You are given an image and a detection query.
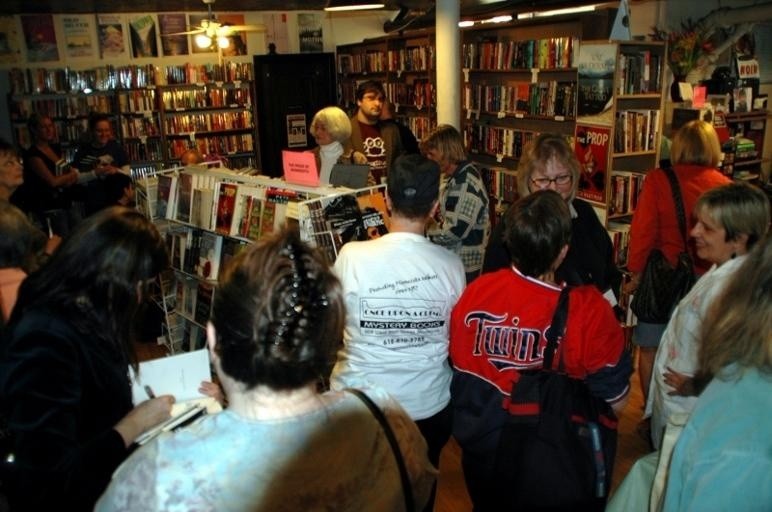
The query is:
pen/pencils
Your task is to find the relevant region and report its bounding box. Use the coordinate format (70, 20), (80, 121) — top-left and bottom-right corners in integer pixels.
(144, 386), (155, 399)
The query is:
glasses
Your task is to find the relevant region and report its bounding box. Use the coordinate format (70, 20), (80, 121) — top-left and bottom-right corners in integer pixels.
(530, 175), (572, 188)
(3, 158), (23, 168)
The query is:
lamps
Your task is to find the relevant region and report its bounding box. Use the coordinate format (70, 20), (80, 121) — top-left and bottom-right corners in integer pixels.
(194, 35), (230, 50)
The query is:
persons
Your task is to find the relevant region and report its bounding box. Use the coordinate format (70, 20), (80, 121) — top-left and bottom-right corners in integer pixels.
(1, 77), (772, 511)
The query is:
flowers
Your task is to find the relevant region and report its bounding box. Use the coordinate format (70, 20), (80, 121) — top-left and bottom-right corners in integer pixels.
(646, 23), (716, 82)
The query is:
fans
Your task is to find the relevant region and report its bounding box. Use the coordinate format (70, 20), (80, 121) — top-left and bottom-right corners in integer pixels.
(158, 1), (268, 36)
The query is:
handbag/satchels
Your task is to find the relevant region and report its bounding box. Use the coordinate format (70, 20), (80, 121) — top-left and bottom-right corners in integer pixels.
(462, 370), (618, 512)
(630, 250), (696, 324)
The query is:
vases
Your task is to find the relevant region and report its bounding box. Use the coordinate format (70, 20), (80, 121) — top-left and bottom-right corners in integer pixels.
(669, 79), (686, 102)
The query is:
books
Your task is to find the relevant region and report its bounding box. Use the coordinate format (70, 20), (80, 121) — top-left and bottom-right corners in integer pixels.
(2, 43), (436, 447)
(460, 37), (772, 371)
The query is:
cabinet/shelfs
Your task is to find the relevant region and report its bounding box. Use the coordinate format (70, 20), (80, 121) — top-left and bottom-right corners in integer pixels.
(136, 159), (392, 354)
(607, 43), (666, 268)
(711, 105), (769, 183)
(0, 84), (256, 166)
(459, 8), (618, 220)
(336, 29), (437, 140)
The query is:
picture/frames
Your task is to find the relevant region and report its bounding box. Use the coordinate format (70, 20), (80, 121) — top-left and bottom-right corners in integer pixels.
(707, 87), (753, 128)
(575, 40), (619, 126)
(573, 123), (611, 209)
